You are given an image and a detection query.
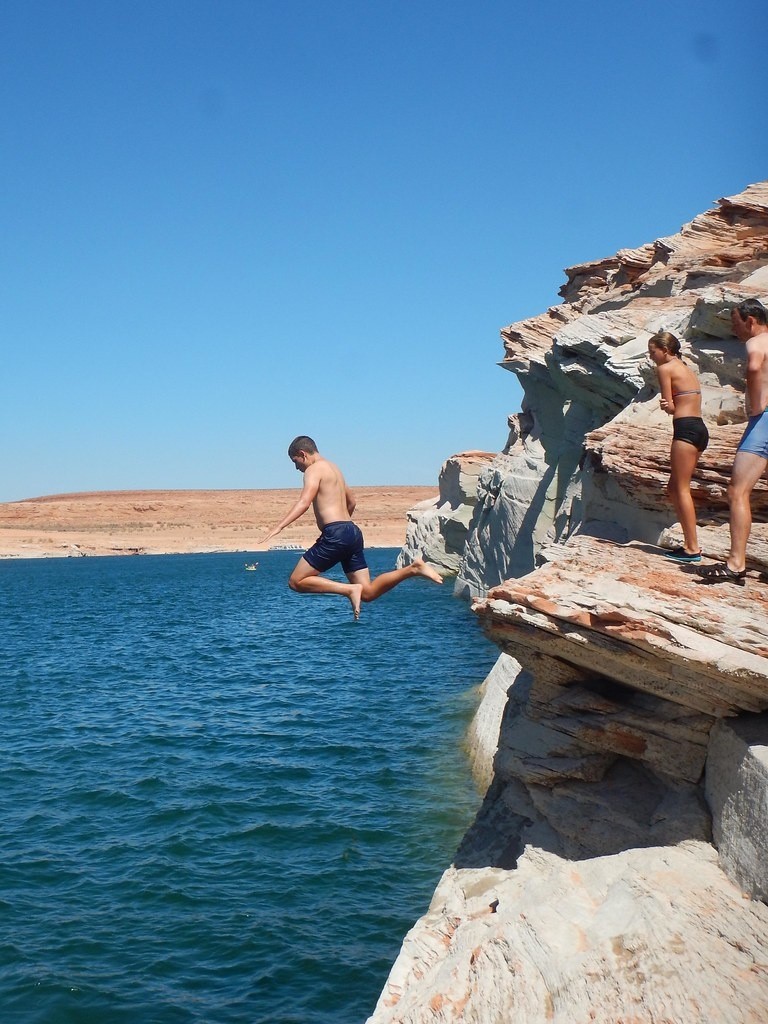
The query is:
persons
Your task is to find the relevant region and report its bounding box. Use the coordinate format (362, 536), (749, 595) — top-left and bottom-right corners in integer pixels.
(694, 299), (768, 585)
(649, 332), (709, 561)
(257, 436), (444, 620)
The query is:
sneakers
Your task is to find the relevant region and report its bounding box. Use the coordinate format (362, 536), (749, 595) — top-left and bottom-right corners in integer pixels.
(663, 547), (702, 561)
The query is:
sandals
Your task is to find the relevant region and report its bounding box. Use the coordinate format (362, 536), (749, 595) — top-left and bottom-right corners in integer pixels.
(696, 563), (746, 586)
